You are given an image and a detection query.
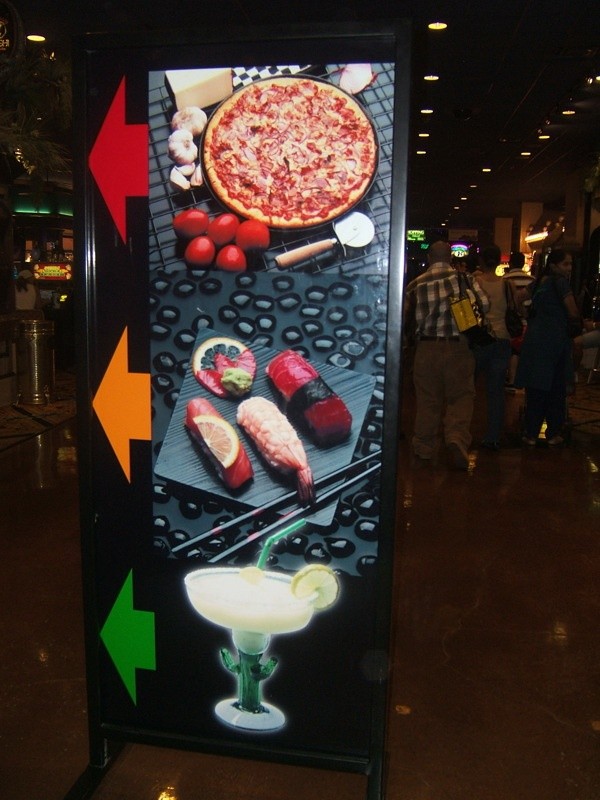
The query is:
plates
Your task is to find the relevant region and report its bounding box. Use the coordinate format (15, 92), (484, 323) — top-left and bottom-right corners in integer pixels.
(199, 74), (381, 234)
(154, 326), (378, 528)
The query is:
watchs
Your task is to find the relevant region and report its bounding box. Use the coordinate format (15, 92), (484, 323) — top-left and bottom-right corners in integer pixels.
(592, 321), (596, 330)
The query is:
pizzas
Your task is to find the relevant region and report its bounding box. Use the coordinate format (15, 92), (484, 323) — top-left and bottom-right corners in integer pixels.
(203, 77), (377, 228)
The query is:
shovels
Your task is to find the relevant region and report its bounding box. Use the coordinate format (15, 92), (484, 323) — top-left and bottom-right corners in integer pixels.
(275, 211), (375, 269)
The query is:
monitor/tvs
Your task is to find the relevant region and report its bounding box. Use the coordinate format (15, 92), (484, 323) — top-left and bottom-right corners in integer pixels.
(450, 241), (481, 258)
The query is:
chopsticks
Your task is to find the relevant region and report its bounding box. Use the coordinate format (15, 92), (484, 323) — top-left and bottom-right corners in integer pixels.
(170, 450), (382, 561)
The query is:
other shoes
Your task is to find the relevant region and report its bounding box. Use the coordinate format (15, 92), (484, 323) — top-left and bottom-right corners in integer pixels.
(548, 435), (578, 449)
(447, 440), (469, 470)
(481, 440), (499, 451)
(414, 444), (434, 460)
(509, 432), (546, 447)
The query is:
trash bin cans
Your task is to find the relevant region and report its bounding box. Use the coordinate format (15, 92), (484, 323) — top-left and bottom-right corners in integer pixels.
(17, 319), (60, 404)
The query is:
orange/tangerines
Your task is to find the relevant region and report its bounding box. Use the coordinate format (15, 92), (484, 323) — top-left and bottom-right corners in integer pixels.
(193, 338), (255, 395)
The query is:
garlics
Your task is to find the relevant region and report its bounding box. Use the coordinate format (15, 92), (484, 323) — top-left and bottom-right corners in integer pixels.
(168, 106), (208, 190)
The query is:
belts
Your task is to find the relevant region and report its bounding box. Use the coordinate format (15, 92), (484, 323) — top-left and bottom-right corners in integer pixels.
(420, 335), (460, 342)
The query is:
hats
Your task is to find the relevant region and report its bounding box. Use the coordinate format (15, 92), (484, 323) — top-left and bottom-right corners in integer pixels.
(18, 270), (33, 279)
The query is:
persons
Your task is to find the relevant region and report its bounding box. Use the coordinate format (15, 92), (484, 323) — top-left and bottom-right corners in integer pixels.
(399, 227), (600, 474)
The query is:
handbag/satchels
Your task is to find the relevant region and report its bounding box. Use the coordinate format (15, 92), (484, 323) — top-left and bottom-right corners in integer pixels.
(450, 272), (497, 347)
(504, 281), (523, 338)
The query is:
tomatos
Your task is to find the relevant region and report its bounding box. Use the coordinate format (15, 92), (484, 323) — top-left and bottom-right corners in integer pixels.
(172, 209), (270, 273)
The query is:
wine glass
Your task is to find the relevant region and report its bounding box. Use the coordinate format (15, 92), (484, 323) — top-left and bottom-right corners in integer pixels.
(184, 568), (320, 735)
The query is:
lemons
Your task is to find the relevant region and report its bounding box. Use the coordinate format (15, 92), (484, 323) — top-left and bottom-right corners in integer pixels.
(292, 565), (339, 610)
(193, 416), (240, 468)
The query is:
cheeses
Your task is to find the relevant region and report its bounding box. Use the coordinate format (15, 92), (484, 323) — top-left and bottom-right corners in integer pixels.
(164, 67), (234, 109)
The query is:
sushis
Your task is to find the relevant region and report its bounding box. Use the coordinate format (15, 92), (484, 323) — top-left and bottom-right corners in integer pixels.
(184, 352), (352, 506)
